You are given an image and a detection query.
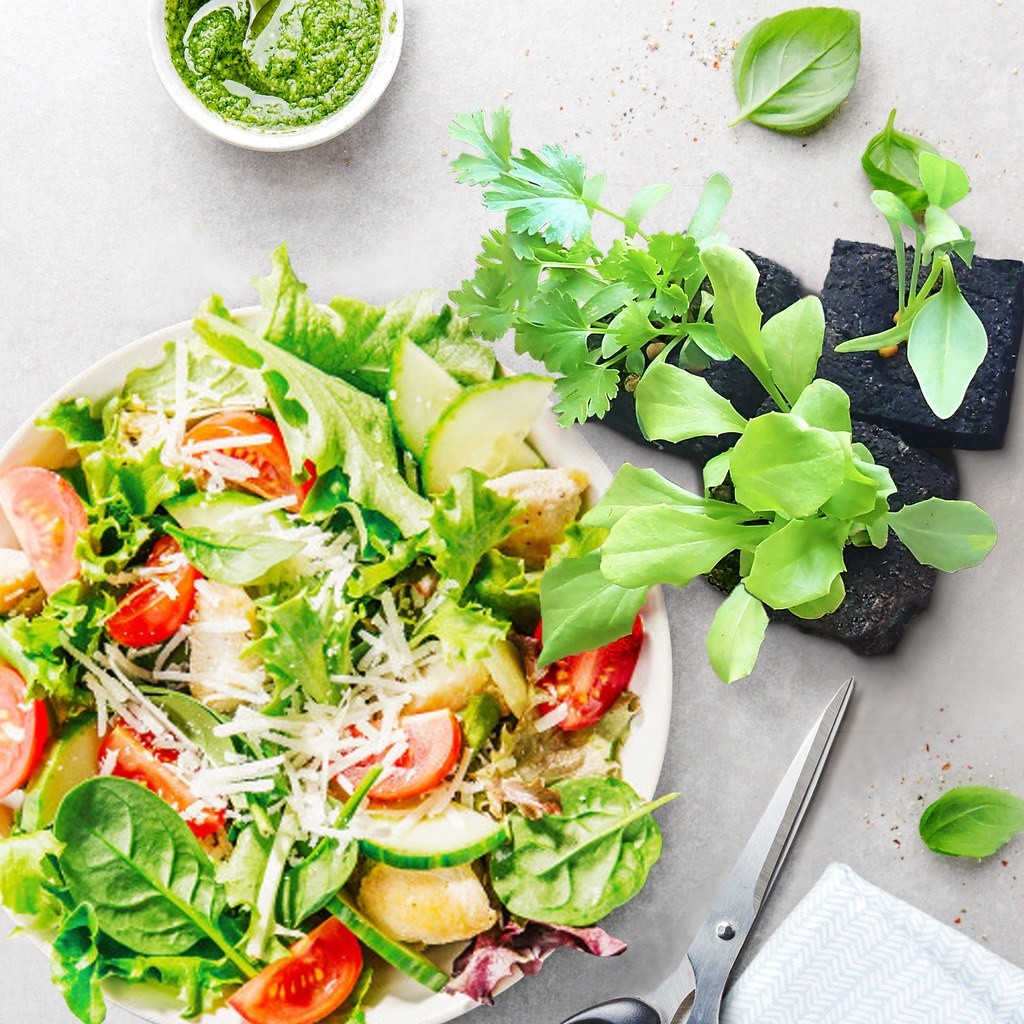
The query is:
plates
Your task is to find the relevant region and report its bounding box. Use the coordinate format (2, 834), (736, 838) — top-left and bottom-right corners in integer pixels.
(3, 303), (673, 1023)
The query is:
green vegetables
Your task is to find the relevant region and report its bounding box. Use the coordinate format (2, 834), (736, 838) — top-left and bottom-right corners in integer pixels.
(2, 251), (672, 1024)
(447, 2), (996, 685)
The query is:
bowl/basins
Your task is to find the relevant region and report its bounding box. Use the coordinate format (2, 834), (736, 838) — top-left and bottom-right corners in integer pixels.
(145, 0), (404, 153)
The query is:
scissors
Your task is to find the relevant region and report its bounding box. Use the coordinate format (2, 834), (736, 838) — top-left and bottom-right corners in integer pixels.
(556, 678), (859, 1024)
